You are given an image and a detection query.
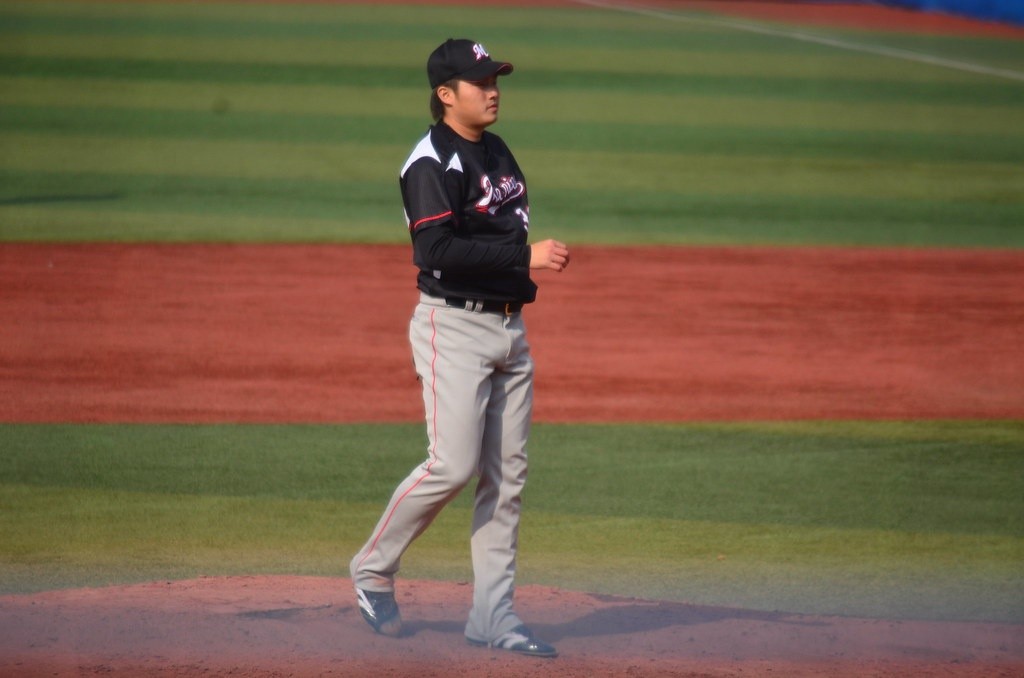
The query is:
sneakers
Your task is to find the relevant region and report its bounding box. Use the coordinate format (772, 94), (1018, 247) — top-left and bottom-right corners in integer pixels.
(467, 622), (560, 656)
(354, 581), (405, 635)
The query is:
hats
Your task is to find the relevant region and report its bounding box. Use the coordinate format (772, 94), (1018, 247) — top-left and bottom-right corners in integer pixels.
(428, 38), (514, 88)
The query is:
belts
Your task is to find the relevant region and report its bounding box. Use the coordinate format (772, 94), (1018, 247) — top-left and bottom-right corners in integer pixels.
(445, 295), (523, 316)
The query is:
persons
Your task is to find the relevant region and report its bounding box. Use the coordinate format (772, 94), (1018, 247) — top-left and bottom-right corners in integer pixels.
(350, 37), (572, 656)
